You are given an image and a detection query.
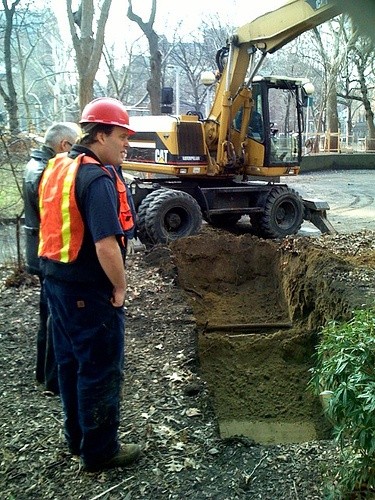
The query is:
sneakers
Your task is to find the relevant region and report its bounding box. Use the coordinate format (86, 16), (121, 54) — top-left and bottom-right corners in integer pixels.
(84, 444), (141, 470)
(70, 447), (78, 453)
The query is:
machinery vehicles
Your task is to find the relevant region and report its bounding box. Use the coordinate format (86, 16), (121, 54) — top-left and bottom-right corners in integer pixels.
(117, 0), (346, 251)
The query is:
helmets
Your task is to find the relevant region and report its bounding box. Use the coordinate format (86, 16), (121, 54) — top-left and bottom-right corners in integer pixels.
(79, 97), (136, 135)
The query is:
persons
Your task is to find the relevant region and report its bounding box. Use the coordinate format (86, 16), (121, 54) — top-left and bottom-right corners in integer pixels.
(22, 122), (82, 398)
(232, 98), (262, 142)
(37, 97), (141, 472)
